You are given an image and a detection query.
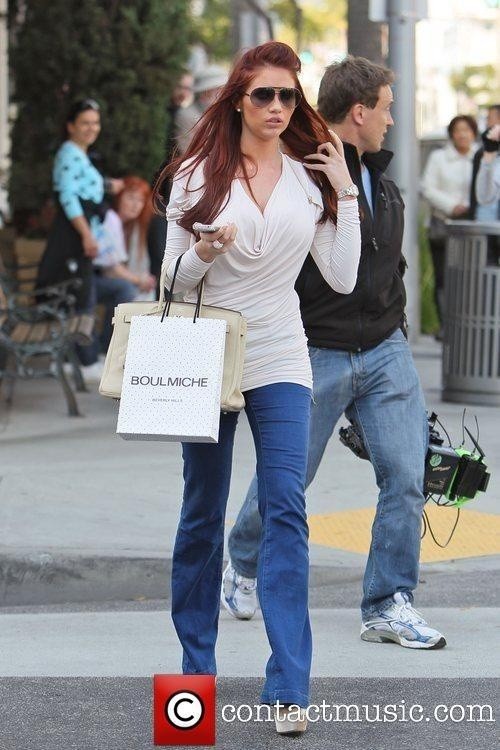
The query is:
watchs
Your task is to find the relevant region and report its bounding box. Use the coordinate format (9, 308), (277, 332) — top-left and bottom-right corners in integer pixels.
(337, 183), (360, 200)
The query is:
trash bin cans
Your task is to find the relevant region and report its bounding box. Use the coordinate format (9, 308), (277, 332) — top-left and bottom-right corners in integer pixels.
(442, 218), (500, 407)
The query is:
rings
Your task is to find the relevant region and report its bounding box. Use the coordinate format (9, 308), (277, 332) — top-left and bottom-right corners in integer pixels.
(212, 239), (223, 250)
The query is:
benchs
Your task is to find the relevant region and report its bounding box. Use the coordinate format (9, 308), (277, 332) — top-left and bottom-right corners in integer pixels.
(0, 258), (94, 417)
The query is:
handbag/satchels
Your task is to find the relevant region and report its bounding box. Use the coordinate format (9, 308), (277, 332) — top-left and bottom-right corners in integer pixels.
(113, 253), (228, 446)
(97, 260), (248, 414)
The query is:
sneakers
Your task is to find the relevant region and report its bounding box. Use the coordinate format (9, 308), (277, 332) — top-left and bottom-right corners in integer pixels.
(358, 590), (447, 650)
(221, 558), (258, 621)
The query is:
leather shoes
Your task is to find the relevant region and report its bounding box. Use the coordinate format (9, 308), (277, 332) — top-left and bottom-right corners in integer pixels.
(272, 703), (309, 734)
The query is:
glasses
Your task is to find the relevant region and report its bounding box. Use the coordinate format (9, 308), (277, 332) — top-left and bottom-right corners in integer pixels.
(241, 84), (304, 113)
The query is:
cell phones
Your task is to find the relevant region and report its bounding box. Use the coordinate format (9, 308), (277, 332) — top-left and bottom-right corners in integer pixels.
(193, 222), (222, 233)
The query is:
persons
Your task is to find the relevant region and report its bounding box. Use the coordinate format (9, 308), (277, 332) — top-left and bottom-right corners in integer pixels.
(420, 116), (482, 339)
(149, 42), (362, 735)
(47, 100), (123, 383)
(467, 104), (500, 272)
(35, 200), (138, 360)
(475, 125), (500, 206)
(217, 53), (445, 649)
(164, 68), (189, 163)
(95, 175), (158, 293)
(175, 66), (228, 159)
